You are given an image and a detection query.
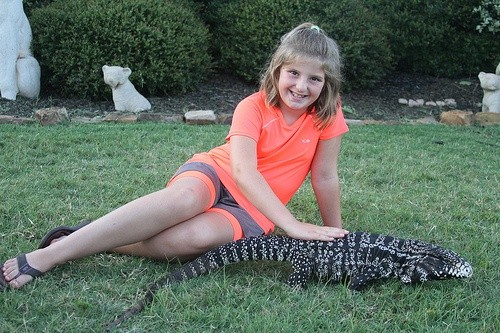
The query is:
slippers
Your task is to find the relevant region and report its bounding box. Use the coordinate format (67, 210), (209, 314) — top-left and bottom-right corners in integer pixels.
(39, 221), (91, 250)
(1, 254), (42, 290)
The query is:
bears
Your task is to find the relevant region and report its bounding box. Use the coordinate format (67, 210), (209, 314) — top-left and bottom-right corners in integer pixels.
(102, 65), (152, 112)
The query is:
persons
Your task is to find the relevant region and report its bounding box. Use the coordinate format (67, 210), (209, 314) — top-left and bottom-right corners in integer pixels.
(1, 23), (350, 293)
